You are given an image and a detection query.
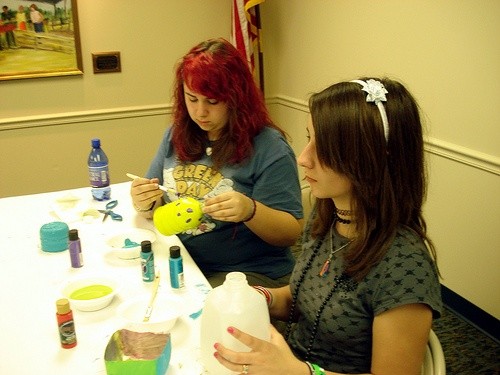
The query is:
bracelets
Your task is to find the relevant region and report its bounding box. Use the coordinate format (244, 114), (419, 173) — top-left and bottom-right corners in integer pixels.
(254, 284), (273, 308)
(231, 198), (257, 236)
(305, 361), (325, 375)
(133, 199), (158, 212)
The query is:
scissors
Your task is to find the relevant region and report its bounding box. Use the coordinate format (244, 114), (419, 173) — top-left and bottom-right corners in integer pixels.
(98, 200), (123, 222)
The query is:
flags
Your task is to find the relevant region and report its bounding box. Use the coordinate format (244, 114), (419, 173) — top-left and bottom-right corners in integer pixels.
(230, 0), (258, 73)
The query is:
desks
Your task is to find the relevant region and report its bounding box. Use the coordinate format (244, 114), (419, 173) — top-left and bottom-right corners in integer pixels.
(0, 180), (213, 375)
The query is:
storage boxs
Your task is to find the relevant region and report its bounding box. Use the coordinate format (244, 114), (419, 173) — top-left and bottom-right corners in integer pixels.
(104, 328), (171, 375)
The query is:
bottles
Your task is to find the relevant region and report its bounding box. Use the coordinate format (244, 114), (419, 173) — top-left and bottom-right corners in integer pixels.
(55, 298), (77, 349)
(169, 246), (184, 289)
(140, 240), (155, 282)
(199, 271), (270, 375)
(68, 229), (84, 268)
(40, 222), (69, 252)
(87, 139), (111, 200)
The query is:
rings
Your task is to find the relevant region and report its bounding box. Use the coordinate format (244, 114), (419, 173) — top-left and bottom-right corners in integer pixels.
(241, 364), (248, 374)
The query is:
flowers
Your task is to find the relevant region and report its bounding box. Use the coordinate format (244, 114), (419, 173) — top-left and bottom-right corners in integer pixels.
(361, 79), (389, 103)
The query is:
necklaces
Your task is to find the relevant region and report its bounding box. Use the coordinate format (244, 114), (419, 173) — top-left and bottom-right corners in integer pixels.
(284, 204), (358, 362)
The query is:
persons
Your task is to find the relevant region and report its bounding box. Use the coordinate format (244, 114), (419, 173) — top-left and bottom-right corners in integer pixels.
(129, 37), (301, 293)
(213, 75), (443, 375)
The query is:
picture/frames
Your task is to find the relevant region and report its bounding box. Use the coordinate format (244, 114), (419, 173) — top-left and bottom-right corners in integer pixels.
(0, 0), (84, 81)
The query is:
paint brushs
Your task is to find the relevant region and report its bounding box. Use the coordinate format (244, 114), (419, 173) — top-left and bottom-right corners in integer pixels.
(143, 270), (159, 322)
(128, 173), (178, 194)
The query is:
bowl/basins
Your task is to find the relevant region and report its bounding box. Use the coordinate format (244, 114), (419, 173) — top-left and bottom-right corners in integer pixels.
(65, 281), (118, 311)
(104, 227), (156, 260)
